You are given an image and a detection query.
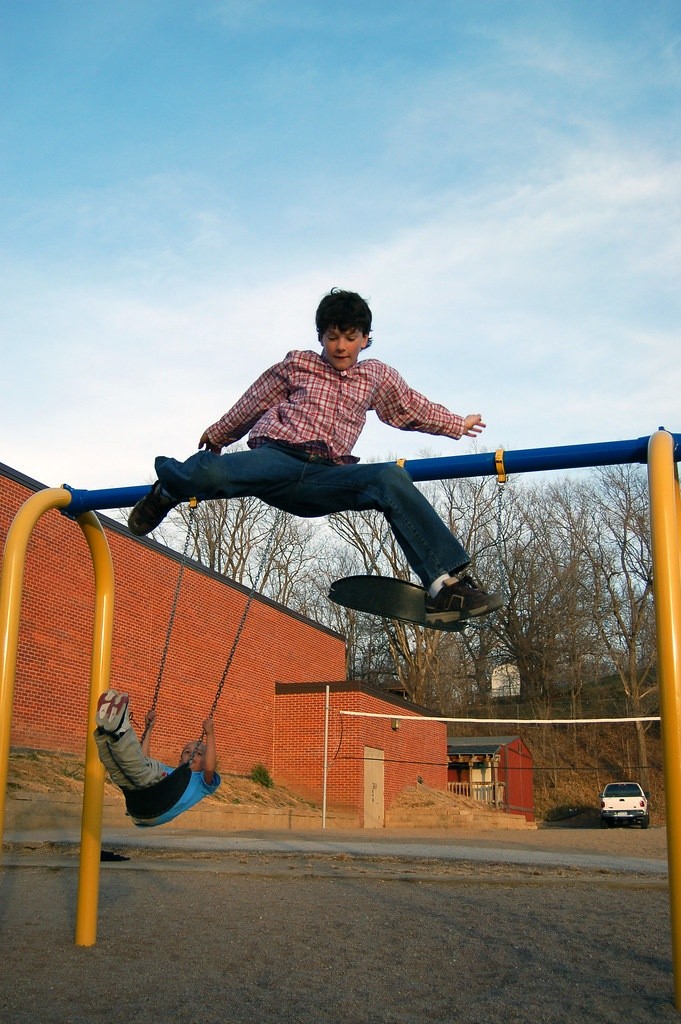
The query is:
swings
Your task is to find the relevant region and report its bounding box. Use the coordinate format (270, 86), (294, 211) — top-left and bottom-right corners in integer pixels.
(118, 506), (282, 820)
(327, 484), (506, 632)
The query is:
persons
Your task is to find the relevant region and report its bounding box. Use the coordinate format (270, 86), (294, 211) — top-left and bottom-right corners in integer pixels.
(93, 689), (220, 827)
(127, 287), (504, 625)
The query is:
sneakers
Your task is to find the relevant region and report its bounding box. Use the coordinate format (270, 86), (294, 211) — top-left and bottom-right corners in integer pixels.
(95, 688), (131, 735)
(128, 480), (180, 537)
(423, 576), (505, 627)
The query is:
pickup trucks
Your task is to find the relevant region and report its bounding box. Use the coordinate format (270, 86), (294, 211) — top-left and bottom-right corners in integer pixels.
(599, 783), (650, 830)
